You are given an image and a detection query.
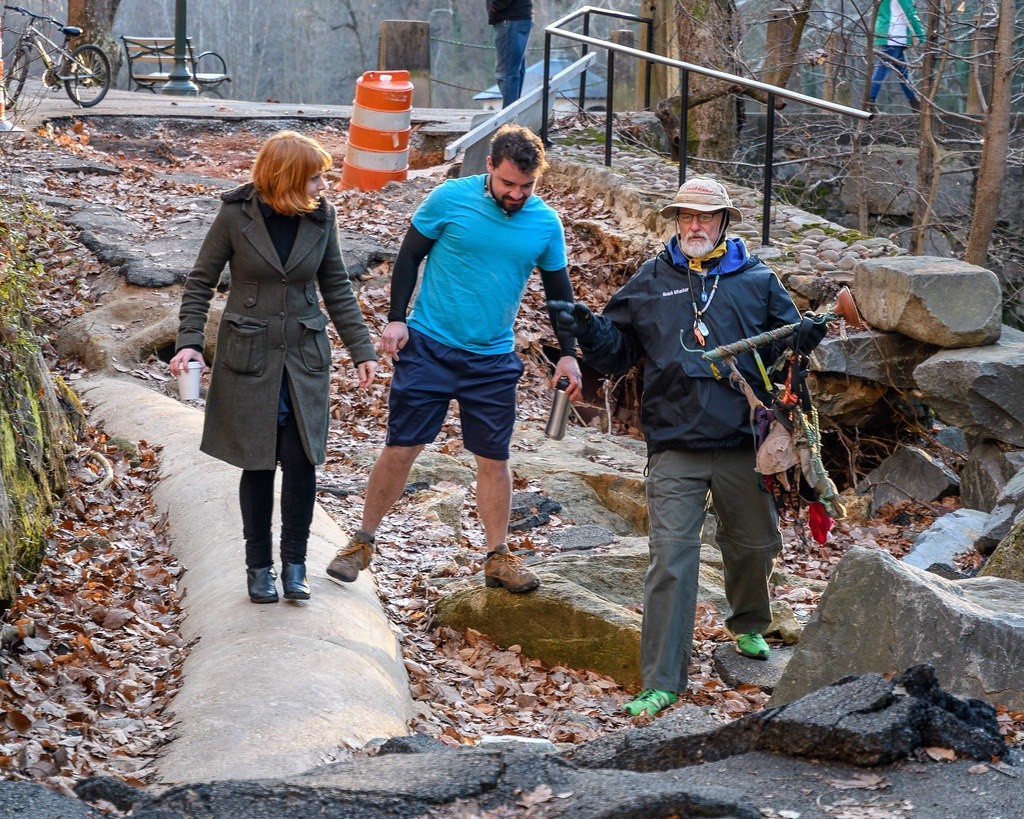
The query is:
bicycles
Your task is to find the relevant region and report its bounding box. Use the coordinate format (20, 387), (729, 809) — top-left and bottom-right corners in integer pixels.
(1, 4), (114, 112)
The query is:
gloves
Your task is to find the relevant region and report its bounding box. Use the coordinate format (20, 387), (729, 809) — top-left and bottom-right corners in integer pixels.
(792, 309), (828, 356)
(547, 300), (601, 338)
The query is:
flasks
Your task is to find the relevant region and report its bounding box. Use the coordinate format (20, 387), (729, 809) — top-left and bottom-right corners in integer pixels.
(544, 380), (576, 440)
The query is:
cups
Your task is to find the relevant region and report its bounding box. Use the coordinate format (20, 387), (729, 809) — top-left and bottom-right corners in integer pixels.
(175, 360), (202, 402)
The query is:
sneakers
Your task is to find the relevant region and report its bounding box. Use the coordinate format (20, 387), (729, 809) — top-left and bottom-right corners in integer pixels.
(483, 544), (540, 593)
(722, 623), (771, 661)
(326, 530), (376, 582)
(620, 687), (678, 720)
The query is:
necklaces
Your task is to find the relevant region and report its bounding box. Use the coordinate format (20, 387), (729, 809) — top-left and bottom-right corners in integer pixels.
(483, 184), (512, 220)
(686, 267), (719, 347)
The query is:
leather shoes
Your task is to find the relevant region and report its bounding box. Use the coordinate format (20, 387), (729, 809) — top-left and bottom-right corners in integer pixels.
(246, 564), (278, 603)
(280, 558), (310, 601)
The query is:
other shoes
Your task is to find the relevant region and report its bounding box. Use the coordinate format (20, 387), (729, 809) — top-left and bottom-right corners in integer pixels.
(909, 97), (921, 114)
(865, 99), (877, 112)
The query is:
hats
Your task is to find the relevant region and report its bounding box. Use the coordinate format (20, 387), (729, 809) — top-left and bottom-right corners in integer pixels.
(658, 177), (743, 228)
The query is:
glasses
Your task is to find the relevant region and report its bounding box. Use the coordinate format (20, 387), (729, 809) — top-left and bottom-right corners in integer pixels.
(675, 209), (725, 223)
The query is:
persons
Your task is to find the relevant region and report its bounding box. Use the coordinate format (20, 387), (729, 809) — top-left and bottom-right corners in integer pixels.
(865, 0), (924, 116)
(171, 128), (380, 604)
(486, 0), (536, 109)
(545, 178), (824, 722)
(327, 123), (583, 593)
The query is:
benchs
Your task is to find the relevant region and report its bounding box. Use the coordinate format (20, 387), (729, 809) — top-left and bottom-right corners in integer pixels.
(118, 35), (233, 100)
